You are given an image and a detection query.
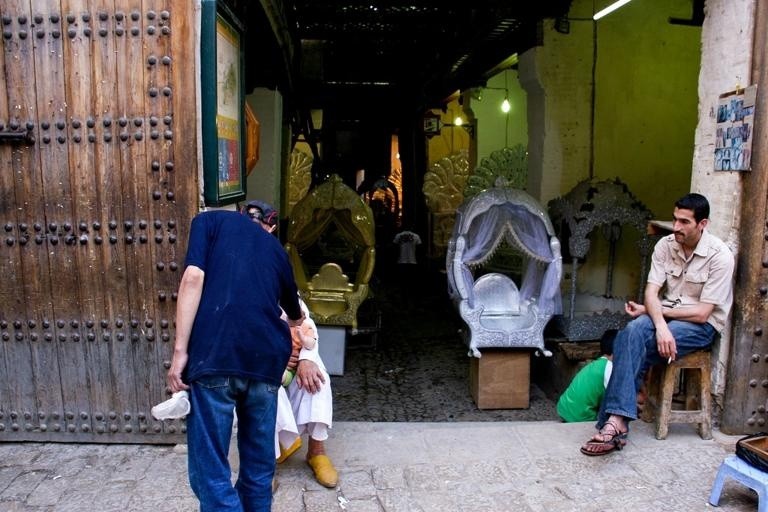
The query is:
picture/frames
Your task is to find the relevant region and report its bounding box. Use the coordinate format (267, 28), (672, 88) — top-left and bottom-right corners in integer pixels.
(201, 0), (247, 208)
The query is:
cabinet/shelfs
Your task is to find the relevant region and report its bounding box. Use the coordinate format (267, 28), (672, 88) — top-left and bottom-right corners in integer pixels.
(316, 325), (346, 378)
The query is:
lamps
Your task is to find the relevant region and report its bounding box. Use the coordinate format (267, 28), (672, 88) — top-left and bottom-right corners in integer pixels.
(455, 68), (510, 126)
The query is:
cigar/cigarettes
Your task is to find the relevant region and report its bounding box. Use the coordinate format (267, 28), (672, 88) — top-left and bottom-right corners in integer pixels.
(668, 355), (672, 364)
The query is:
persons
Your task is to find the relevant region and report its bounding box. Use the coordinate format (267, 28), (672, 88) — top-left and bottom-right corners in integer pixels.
(164, 197), (306, 512)
(708, 97), (753, 171)
(270, 297), (339, 490)
(578, 191), (736, 460)
(556, 328), (619, 423)
(392, 224), (424, 311)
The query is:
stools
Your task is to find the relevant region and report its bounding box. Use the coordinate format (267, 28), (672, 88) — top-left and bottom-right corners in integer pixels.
(644, 347), (768, 512)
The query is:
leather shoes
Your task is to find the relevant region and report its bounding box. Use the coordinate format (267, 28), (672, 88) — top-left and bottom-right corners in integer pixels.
(306, 446), (338, 489)
(275, 436), (302, 464)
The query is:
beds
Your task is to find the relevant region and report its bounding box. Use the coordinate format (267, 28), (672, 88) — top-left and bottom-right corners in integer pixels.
(547, 175), (651, 343)
(424, 145), (530, 274)
(445, 187), (561, 355)
(282, 178), (376, 338)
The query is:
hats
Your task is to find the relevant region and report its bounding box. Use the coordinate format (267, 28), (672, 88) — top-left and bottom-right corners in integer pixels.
(241, 200), (279, 226)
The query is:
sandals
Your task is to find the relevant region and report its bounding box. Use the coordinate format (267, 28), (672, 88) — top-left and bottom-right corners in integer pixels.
(580, 413), (629, 456)
